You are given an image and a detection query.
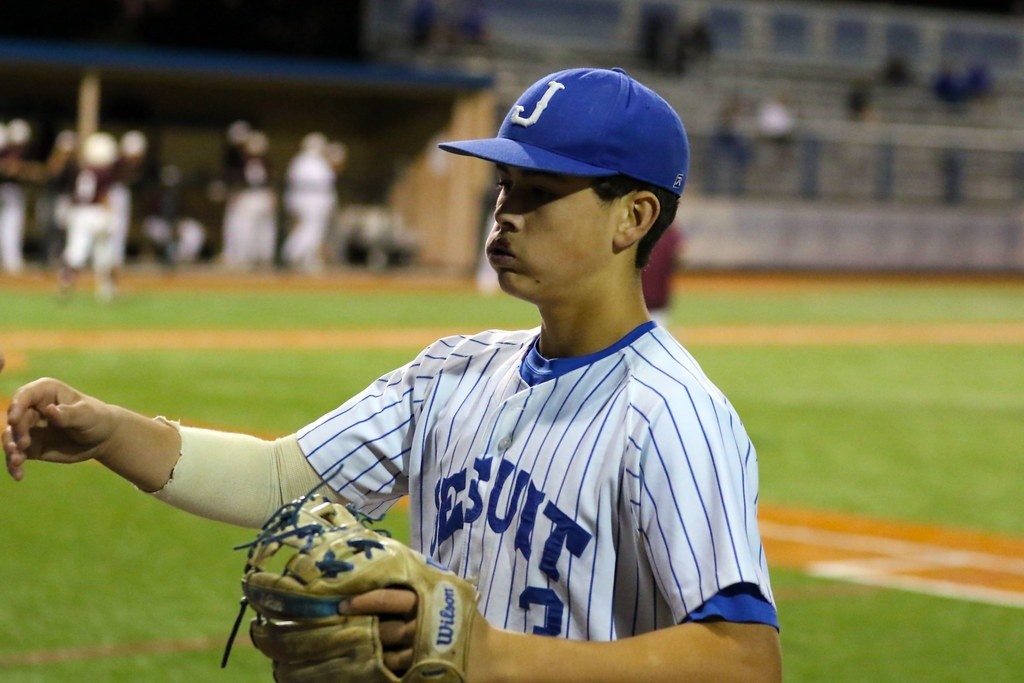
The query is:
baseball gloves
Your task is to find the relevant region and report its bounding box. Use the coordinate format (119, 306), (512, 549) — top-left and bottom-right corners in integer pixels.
(241, 491), (483, 683)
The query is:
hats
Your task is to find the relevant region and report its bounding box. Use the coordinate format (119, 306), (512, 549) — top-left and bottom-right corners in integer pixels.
(437, 66), (690, 199)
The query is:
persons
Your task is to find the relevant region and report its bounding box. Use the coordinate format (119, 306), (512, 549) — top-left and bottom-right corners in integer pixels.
(0, 117), (409, 303)
(649, 11), (998, 201)
(0, 65), (786, 683)
